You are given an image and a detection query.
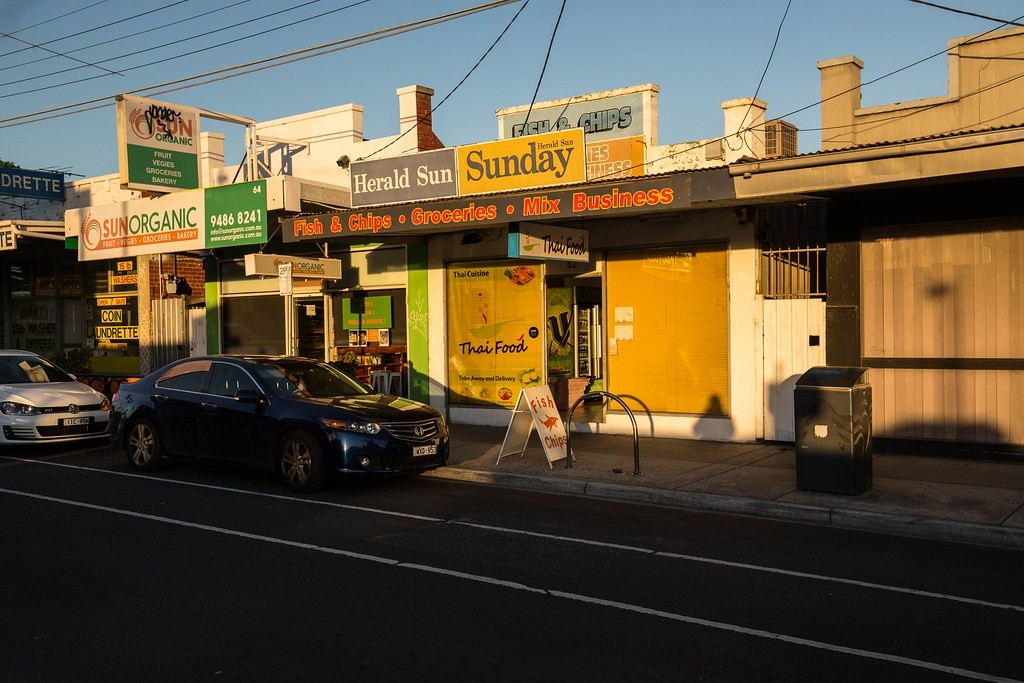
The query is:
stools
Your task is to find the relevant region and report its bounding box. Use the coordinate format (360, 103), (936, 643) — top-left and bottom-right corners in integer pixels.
(371, 370), (402, 397)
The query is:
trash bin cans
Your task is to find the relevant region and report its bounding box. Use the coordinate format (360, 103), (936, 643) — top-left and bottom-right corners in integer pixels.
(792, 365), (873, 496)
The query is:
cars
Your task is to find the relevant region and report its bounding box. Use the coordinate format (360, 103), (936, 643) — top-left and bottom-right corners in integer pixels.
(0, 350), (112, 450)
(105, 353), (450, 492)
